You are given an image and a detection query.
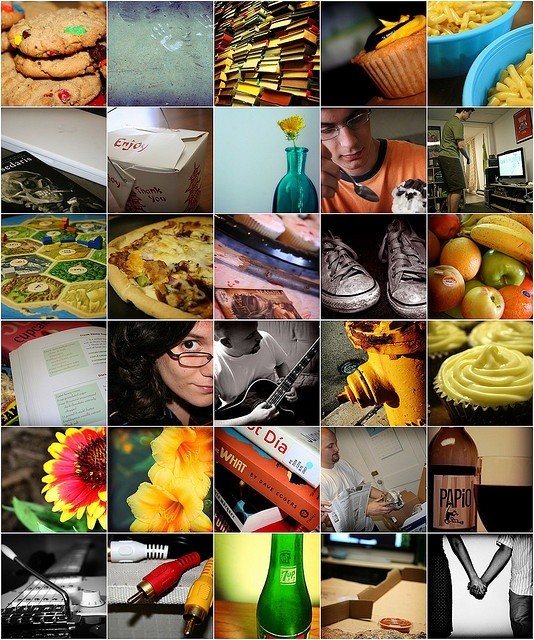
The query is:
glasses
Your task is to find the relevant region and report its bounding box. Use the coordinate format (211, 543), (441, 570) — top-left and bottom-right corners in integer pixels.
(164, 348), (213, 368)
(321, 108), (372, 141)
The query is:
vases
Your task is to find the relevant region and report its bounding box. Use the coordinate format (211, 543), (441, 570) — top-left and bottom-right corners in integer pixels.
(271, 147), (319, 214)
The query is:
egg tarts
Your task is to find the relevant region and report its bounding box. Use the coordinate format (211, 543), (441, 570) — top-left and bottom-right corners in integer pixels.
(379, 618), (412, 633)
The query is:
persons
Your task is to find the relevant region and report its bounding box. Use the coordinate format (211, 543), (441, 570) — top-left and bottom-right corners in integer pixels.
(320, 428), (396, 533)
(321, 106), (427, 215)
(321, 501), (332, 529)
(428, 534), (487, 639)
(108, 321), (215, 426)
(465, 533), (532, 637)
(2, 150), (109, 213)
(432, 108), (475, 211)
(214, 323), (318, 426)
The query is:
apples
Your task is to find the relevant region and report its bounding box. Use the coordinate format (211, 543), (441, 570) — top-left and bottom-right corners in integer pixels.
(449, 280), (482, 319)
(431, 216), (460, 235)
(429, 230), (441, 265)
(428, 263), (464, 313)
(481, 249), (526, 289)
(461, 287), (505, 320)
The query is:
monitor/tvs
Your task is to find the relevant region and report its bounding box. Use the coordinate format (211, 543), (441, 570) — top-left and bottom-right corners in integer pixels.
(497, 147), (529, 186)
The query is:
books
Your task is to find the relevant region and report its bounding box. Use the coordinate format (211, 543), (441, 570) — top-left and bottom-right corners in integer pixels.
(1, 322), (106, 426)
(214, 427), (320, 532)
(214, 0), (320, 107)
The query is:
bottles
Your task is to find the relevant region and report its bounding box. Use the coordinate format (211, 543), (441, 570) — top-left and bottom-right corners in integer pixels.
(256, 534), (312, 639)
(428, 427), (478, 532)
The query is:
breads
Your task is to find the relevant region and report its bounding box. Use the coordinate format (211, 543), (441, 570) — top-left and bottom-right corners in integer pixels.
(281, 214), (320, 252)
(233, 214), (285, 240)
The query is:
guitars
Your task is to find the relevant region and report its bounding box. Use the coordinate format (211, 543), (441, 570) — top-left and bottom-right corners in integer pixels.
(215, 337), (320, 426)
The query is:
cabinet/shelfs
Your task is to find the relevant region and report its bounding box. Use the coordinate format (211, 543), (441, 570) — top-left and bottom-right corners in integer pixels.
(487, 181), (532, 212)
(427, 157), (448, 213)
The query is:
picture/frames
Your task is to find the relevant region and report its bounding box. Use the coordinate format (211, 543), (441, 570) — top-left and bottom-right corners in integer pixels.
(427, 126), (442, 152)
(513, 109), (533, 144)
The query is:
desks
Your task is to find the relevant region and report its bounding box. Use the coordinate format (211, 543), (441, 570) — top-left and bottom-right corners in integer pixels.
(321, 546), (422, 571)
(214, 599), (320, 640)
(321, 576), (374, 608)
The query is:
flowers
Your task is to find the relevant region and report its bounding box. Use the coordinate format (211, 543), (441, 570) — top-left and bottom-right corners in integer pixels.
(277, 113), (306, 197)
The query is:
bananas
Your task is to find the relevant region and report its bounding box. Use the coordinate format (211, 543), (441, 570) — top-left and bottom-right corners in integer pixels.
(458, 213), (533, 277)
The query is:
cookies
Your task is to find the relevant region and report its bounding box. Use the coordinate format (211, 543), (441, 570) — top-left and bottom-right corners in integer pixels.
(2, 3), (106, 106)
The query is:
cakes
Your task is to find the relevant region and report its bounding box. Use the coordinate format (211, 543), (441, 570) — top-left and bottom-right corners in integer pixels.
(391, 179), (426, 214)
(427, 323), (466, 361)
(431, 345), (533, 423)
(467, 321), (532, 354)
(353, 13), (425, 100)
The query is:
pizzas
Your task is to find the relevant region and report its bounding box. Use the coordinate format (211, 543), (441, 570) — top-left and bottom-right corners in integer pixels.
(322, 628), (424, 638)
(108, 216), (213, 319)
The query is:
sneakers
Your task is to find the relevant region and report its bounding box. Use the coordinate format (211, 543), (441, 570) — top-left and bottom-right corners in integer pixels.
(322, 230), (381, 313)
(377, 219), (426, 319)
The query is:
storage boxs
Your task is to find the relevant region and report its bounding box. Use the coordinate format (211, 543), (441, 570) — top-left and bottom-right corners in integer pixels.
(107, 126), (208, 213)
(383, 489), (422, 530)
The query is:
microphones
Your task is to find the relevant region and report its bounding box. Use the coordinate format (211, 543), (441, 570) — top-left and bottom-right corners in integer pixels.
(1, 543), (80, 630)
